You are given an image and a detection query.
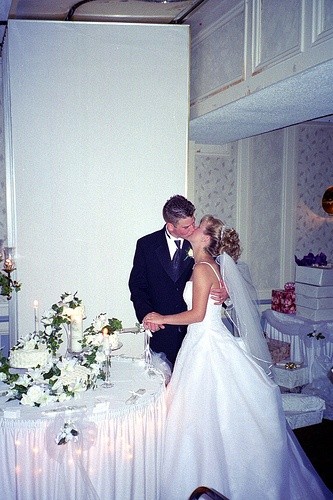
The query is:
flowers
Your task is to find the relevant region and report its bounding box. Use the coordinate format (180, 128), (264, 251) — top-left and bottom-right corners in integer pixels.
(0, 294), (123, 407)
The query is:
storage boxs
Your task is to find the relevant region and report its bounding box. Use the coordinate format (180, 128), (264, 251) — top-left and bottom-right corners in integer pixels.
(271, 265), (333, 322)
(281, 393), (325, 430)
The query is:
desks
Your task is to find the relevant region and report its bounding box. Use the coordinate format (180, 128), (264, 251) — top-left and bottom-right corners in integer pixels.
(0, 356), (166, 500)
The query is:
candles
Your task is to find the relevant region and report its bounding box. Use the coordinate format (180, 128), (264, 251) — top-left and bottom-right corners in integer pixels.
(103, 328), (111, 356)
(70, 307), (83, 352)
(33, 300), (39, 332)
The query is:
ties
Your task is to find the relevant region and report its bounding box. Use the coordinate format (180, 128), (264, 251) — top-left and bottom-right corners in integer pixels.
(166, 230), (181, 269)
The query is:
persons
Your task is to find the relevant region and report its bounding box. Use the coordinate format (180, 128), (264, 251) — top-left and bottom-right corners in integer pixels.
(128, 193), (230, 373)
(141, 214), (333, 500)
